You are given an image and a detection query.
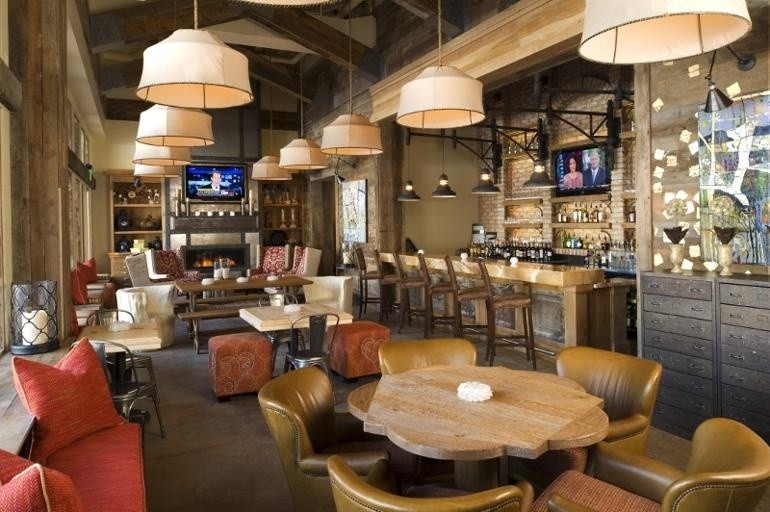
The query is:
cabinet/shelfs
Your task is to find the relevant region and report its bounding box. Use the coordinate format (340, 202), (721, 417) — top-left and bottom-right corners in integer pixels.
(504, 192), (636, 256)
(258, 180), (306, 266)
(107, 172), (166, 287)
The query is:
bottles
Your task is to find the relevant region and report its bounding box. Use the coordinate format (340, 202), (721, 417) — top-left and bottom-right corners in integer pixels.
(585, 253), (636, 270)
(593, 206), (599, 223)
(470, 241), (496, 259)
(579, 209), (582, 222)
(588, 204), (593, 222)
(582, 211), (588, 223)
(563, 232), (636, 251)
(561, 209), (568, 223)
(598, 204), (604, 223)
(495, 238), (553, 263)
(573, 209), (578, 222)
(629, 206), (635, 223)
(557, 209), (562, 222)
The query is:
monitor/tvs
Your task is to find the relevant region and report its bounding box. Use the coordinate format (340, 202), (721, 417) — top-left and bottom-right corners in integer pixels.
(183, 164), (248, 203)
(554, 142), (607, 196)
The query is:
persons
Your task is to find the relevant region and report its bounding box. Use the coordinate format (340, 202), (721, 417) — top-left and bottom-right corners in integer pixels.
(200, 170), (228, 194)
(583, 152), (604, 186)
(562, 155), (583, 189)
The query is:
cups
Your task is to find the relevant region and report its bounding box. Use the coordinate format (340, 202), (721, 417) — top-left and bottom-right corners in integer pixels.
(214, 259), (221, 280)
(269, 294), (284, 310)
(220, 259), (229, 278)
